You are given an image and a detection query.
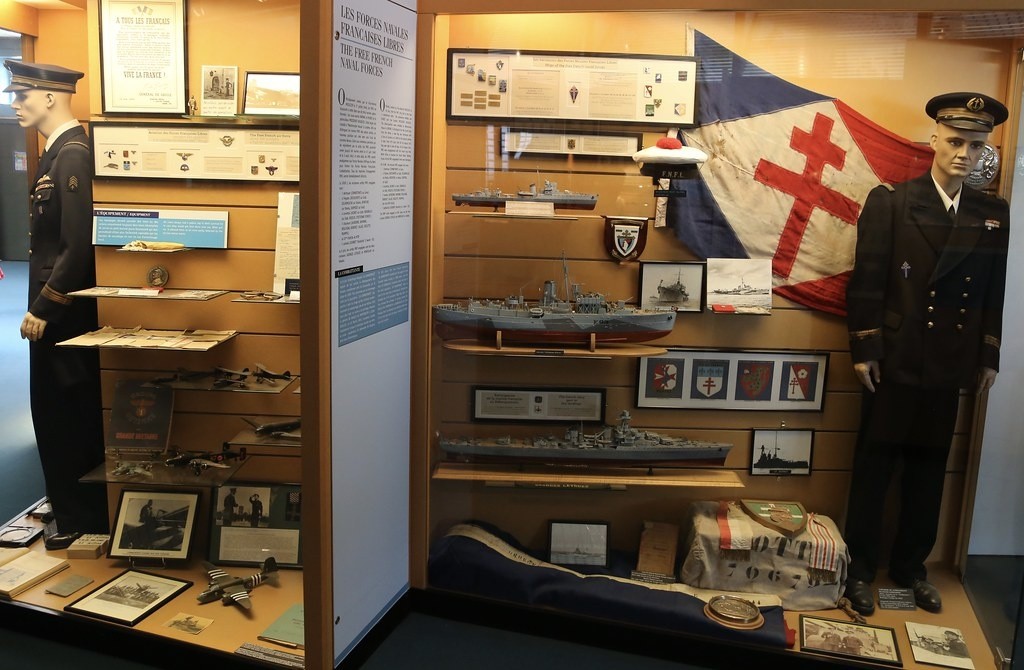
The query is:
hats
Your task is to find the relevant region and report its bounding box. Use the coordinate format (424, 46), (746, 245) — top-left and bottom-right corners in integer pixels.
(925, 92), (1009, 132)
(1, 58), (84, 93)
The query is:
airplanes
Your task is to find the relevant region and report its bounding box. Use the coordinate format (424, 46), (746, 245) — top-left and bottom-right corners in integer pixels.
(212, 362), (292, 389)
(109, 442), (247, 478)
(240, 416), (302, 438)
(197, 557), (279, 610)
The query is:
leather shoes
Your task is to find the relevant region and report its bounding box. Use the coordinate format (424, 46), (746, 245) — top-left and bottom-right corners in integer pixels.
(46, 528), (85, 549)
(842, 575), (874, 615)
(893, 575), (940, 610)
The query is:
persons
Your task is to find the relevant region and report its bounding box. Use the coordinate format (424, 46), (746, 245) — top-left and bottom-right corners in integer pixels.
(821, 627), (841, 652)
(248, 493), (263, 528)
(841, 630), (863, 654)
(838, 90), (1011, 615)
(942, 630), (970, 658)
(139, 499), (155, 523)
(2, 58), (104, 549)
(223, 487), (238, 528)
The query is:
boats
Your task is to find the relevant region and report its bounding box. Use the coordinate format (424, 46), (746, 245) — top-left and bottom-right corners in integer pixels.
(714, 276), (770, 295)
(657, 268), (689, 304)
(754, 431), (808, 468)
(451, 166), (599, 210)
(435, 410), (735, 465)
(432, 249), (678, 346)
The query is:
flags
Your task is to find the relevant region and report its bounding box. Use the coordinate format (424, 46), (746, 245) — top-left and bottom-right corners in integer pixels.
(653, 22), (933, 320)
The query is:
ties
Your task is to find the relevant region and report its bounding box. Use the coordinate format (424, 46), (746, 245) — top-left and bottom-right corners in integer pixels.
(949, 206), (956, 225)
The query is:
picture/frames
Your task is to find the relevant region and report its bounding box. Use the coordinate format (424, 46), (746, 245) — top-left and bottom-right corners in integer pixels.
(500, 128), (644, 162)
(471, 385), (606, 425)
(105, 486), (202, 561)
(97, 0), (188, 116)
(749, 427), (814, 477)
(636, 260), (708, 314)
(210, 479), (303, 570)
(634, 344), (830, 413)
(87, 121), (300, 184)
(446, 47), (697, 128)
(63, 568), (194, 626)
(798, 611), (903, 666)
(547, 519), (610, 569)
(241, 72), (301, 117)
